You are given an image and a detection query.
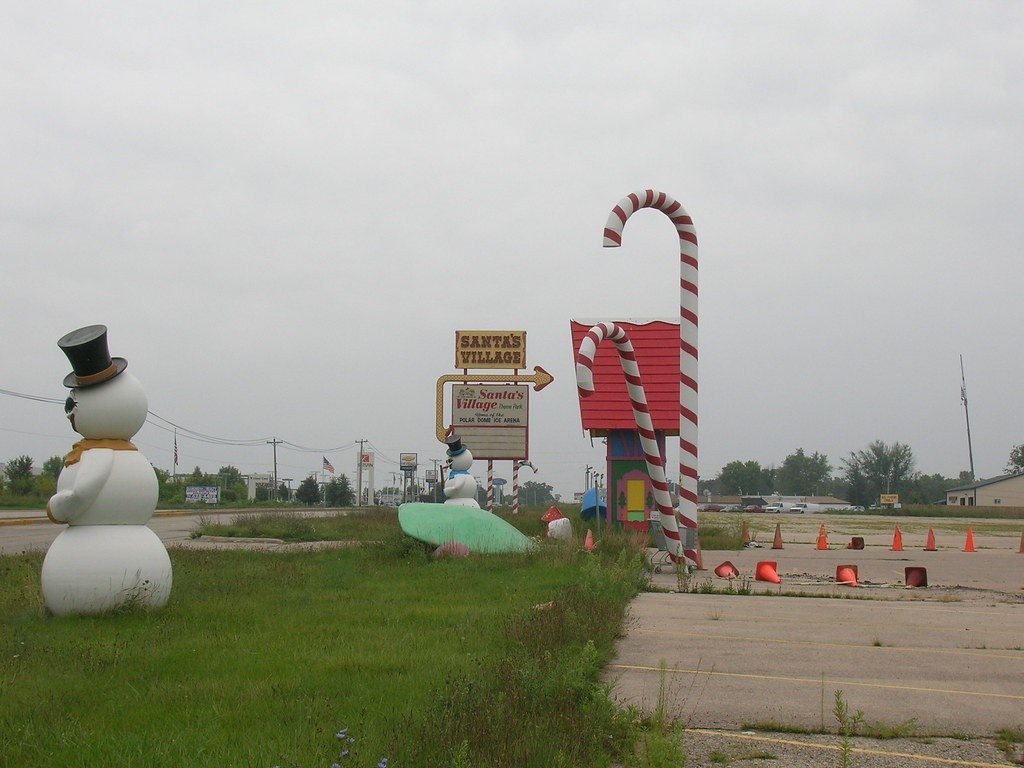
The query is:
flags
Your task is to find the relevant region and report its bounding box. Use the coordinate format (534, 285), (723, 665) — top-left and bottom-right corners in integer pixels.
(322, 456), (335, 475)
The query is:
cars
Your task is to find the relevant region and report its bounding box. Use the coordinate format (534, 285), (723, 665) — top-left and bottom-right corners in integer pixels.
(743, 504), (765, 513)
(702, 504), (720, 512)
(841, 506), (865, 512)
(720, 505), (741, 512)
(789, 503), (808, 514)
(765, 501), (784, 514)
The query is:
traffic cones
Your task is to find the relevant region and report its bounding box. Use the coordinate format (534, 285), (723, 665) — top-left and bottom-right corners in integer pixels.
(772, 523), (785, 550)
(904, 567), (928, 588)
(742, 520), (750, 547)
(813, 521), (834, 550)
(584, 528), (593, 552)
(847, 536), (865, 550)
(835, 564), (859, 588)
(754, 561), (781, 584)
(889, 526), (905, 551)
(923, 527), (938, 551)
(715, 560), (740, 581)
(961, 527), (978, 552)
(1015, 527), (1024, 553)
